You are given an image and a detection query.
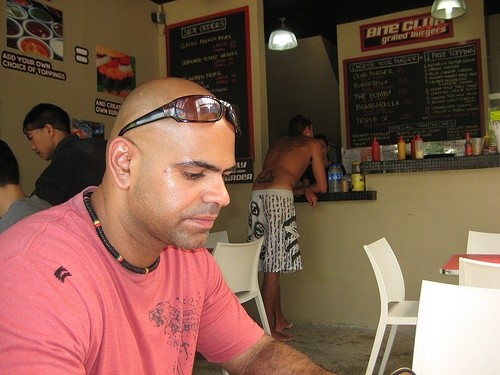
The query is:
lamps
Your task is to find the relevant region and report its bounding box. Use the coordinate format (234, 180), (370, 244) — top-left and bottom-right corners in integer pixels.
(267, 17), (298, 51)
(430, 0), (467, 20)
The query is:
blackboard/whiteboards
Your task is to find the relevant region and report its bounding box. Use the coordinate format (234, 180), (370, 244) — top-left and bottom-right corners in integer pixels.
(341, 37), (486, 150)
(163, 5), (254, 167)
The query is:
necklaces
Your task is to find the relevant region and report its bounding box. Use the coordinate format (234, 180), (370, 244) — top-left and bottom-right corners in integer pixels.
(82, 190), (162, 274)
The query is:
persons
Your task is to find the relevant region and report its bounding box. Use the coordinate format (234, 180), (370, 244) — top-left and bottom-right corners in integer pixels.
(1, 139), (57, 242)
(19, 101), (109, 204)
(301, 134), (349, 192)
(243, 115), (330, 341)
(0, 75), (345, 375)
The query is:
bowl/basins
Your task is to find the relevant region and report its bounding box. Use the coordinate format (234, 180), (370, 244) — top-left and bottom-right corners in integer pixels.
(5, 2), (63, 61)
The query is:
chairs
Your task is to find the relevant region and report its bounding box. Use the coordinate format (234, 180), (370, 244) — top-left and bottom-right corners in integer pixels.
(363, 236), (419, 375)
(212, 237), (272, 375)
(412, 231), (500, 375)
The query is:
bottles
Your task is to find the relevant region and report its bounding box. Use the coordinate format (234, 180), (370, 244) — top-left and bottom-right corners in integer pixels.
(411, 136), (417, 159)
(328, 162), (341, 192)
(414, 134), (424, 159)
(465, 132), (472, 156)
(372, 138), (380, 161)
(484, 132), (490, 149)
(398, 136), (406, 160)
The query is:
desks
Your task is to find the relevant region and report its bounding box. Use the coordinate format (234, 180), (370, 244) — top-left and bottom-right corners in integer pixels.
(437, 253), (500, 276)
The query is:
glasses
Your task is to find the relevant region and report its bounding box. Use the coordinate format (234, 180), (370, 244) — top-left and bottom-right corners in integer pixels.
(118, 94), (242, 139)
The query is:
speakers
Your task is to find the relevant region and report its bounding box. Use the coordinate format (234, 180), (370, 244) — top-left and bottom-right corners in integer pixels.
(152, 12), (164, 24)
